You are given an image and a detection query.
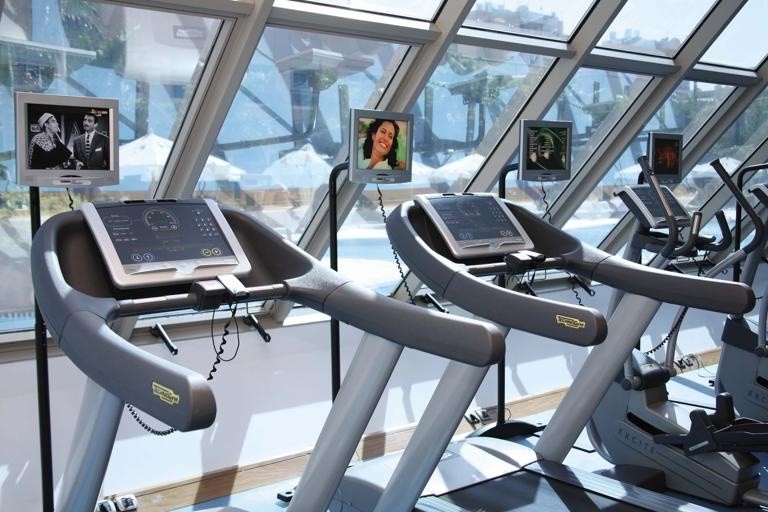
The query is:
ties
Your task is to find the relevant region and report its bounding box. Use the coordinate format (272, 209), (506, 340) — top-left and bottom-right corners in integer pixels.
(85, 134), (91, 158)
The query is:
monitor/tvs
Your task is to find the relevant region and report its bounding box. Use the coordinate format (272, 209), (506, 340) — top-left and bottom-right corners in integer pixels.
(650, 133), (683, 180)
(414, 191), (534, 260)
(14, 91), (119, 189)
(519, 120), (573, 182)
(612, 185), (695, 230)
(349, 107), (414, 183)
(75, 200), (251, 289)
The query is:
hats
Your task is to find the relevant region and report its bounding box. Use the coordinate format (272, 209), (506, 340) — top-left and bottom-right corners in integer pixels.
(38, 113), (53, 128)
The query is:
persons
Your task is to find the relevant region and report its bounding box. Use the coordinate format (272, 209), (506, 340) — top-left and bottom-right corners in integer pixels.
(72, 112), (110, 170)
(28, 111), (72, 169)
(361, 119), (400, 170)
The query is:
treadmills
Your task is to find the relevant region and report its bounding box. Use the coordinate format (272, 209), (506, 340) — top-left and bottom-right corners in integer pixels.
(29, 184), (501, 512)
(387, 192), (768, 511)
